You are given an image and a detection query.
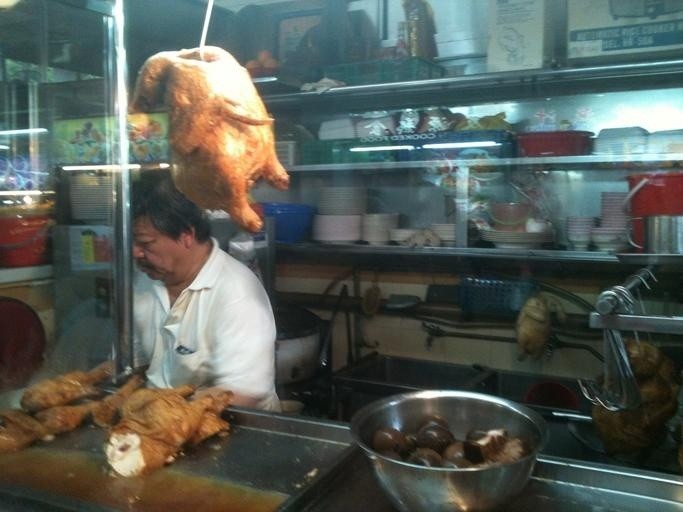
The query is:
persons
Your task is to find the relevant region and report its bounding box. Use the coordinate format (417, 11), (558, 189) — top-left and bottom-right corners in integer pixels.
(108, 167), (282, 414)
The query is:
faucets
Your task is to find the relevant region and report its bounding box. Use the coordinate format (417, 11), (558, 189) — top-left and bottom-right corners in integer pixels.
(542, 332), (558, 362)
(420, 320), (442, 350)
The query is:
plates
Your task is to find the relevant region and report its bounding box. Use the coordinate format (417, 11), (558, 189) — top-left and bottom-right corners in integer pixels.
(387, 228), (422, 245)
(430, 221), (455, 246)
(360, 213), (399, 246)
(599, 192), (629, 229)
(310, 185), (365, 243)
(483, 230), (548, 250)
(68, 173), (111, 224)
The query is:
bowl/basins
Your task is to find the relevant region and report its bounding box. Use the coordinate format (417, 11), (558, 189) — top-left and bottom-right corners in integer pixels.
(564, 216), (599, 251)
(347, 388), (550, 512)
(590, 226), (630, 252)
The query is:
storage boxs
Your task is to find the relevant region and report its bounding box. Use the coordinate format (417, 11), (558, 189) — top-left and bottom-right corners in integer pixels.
(257, 201), (316, 242)
(313, 126), (593, 167)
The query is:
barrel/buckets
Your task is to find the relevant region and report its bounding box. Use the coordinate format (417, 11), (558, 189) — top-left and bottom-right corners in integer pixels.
(617, 173), (683, 252)
(1, 216), (52, 266)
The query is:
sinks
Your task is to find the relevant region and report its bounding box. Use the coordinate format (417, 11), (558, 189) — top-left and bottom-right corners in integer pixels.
(331, 353), (482, 395)
(470, 365), (602, 417)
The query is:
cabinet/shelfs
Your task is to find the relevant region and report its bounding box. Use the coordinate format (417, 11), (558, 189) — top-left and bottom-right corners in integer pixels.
(260, 61), (681, 270)
